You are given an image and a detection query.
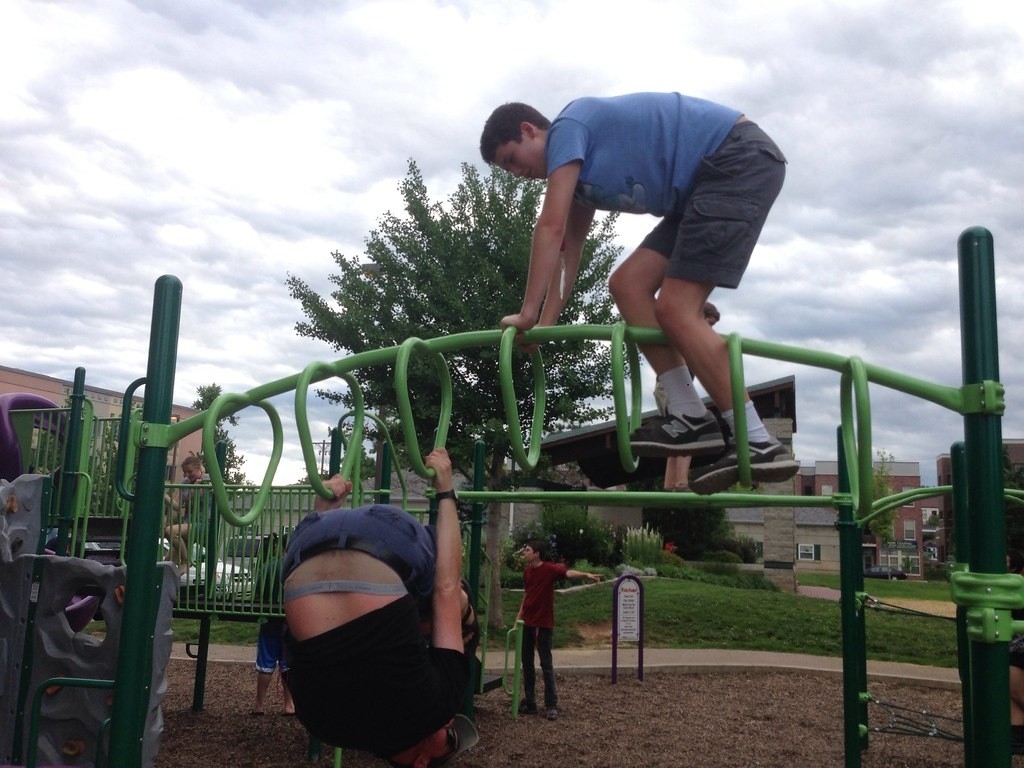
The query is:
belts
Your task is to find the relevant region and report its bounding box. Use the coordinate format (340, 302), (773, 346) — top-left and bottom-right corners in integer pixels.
(283, 538), (421, 596)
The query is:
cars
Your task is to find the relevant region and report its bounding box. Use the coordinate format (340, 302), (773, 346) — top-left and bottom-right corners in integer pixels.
(864, 565), (907, 581)
(217, 537), (263, 569)
(85, 541), (254, 597)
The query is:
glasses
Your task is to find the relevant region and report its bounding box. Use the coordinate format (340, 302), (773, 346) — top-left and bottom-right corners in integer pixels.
(446, 723), (457, 750)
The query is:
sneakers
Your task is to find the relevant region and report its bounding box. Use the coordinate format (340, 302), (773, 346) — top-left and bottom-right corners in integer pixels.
(629, 412), (725, 457)
(518, 699), (538, 713)
(547, 707), (557, 719)
(688, 438), (800, 493)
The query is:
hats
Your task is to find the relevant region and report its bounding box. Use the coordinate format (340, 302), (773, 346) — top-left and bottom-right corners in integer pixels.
(392, 713), (480, 767)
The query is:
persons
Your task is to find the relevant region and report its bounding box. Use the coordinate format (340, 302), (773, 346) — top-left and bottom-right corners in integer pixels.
(163, 456), (225, 577)
(513, 541), (605, 720)
(280, 447), (480, 768)
(1005, 547), (1024, 756)
(252, 532), (294, 717)
(665, 540), (679, 553)
(29, 463), (85, 558)
(480, 94), (801, 495)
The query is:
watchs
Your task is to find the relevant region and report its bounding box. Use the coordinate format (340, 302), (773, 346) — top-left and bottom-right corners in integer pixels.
(436, 488), (457, 502)
